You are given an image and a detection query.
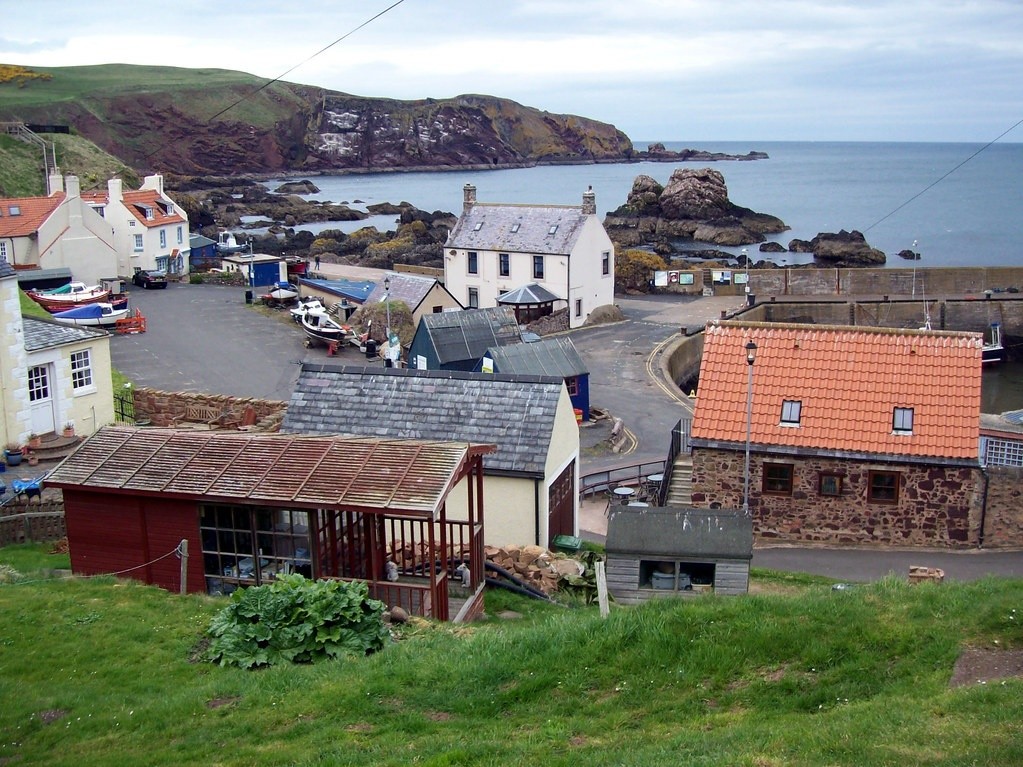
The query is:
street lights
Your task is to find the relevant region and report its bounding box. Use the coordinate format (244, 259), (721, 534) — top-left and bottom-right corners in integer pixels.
(739, 338), (760, 511)
(248, 234), (255, 305)
(742, 248), (749, 306)
(911, 240), (917, 294)
(383, 277), (394, 348)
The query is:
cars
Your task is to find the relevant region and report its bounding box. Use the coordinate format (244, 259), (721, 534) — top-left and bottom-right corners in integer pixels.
(131, 270), (168, 289)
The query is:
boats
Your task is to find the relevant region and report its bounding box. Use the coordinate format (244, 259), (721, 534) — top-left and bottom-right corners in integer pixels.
(300, 303), (349, 348)
(26, 279), (128, 314)
(286, 256), (311, 274)
(50, 301), (130, 326)
(216, 230), (248, 253)
(919, 314), (1005, 364)
(290, 299), (326, 316)
